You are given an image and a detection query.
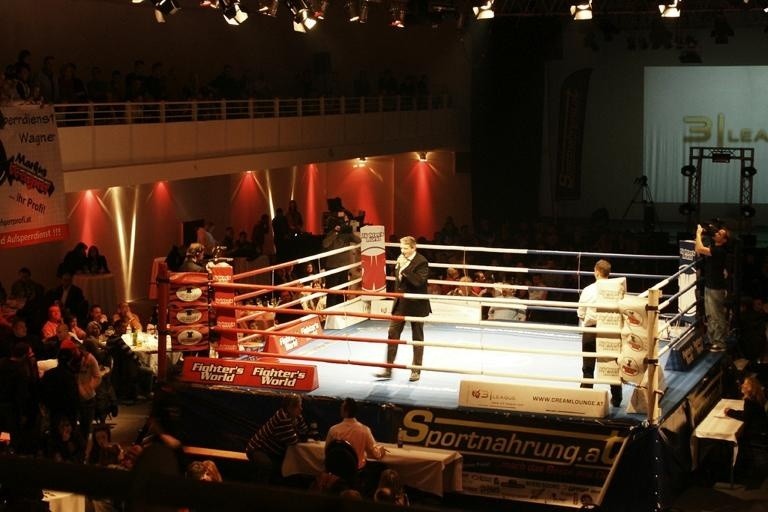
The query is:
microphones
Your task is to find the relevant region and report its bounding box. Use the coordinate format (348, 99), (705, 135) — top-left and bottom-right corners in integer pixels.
(395, 253), (405, 269)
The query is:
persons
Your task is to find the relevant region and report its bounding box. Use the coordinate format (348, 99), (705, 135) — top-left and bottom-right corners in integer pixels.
(696, 223), (732, 351)
(724, 377), (768, 479)
(289, 69), (429, 110)
(738, 251), (768, 360)
(1, 243), (410, 512)
(577, 260), (623, 408)
(372, 236), (432, 381)
(1, 49), (273, 127)
(385, 207), (613, 326)
(166, 198), (375, 309)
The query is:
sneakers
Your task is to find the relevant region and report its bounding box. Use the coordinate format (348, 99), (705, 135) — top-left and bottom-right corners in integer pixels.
(409, 371), (420, 381)
(708, 344), (727, 353)
(375, 370), (392, 378)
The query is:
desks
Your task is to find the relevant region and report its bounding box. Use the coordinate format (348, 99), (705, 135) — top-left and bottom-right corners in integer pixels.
(685, 396), (747, 491)
(277, 435), (468, 505)
(0, 231), (326, 512)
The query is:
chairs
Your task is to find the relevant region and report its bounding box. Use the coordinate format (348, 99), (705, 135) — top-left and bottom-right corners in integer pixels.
(320, 437), (362, 490)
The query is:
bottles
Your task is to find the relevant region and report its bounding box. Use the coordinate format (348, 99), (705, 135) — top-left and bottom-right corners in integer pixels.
(126, 324), (131, 333)
(397, 427), (404, 447)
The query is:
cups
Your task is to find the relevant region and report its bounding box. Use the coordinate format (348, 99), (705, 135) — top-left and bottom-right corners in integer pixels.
(136, 339), (142, 346)
(133, 330), (137, 346)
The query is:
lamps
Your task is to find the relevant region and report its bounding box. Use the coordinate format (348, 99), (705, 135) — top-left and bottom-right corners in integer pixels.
(259, 1), (281, 15)
(569, 3), (594, 20)
(131, 0), (181, 24)
(349, 0), (369, 24)
(387, 5), (406, 29)
(287, 2), (326, 33)
(202, 0), (248, 25)
(472, 1), (497, 20)
